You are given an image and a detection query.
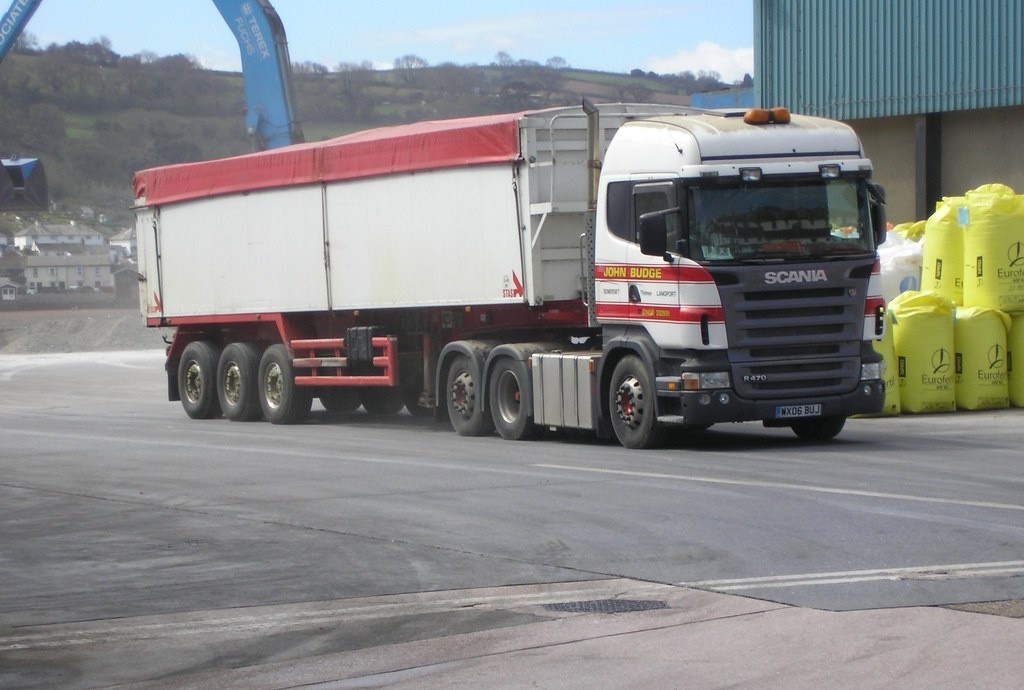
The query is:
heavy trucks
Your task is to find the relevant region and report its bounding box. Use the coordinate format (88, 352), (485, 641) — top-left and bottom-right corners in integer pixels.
(129, 96), (887, 449)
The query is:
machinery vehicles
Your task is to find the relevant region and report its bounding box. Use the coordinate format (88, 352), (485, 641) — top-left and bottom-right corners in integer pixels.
(0, 0), (306, 213)
